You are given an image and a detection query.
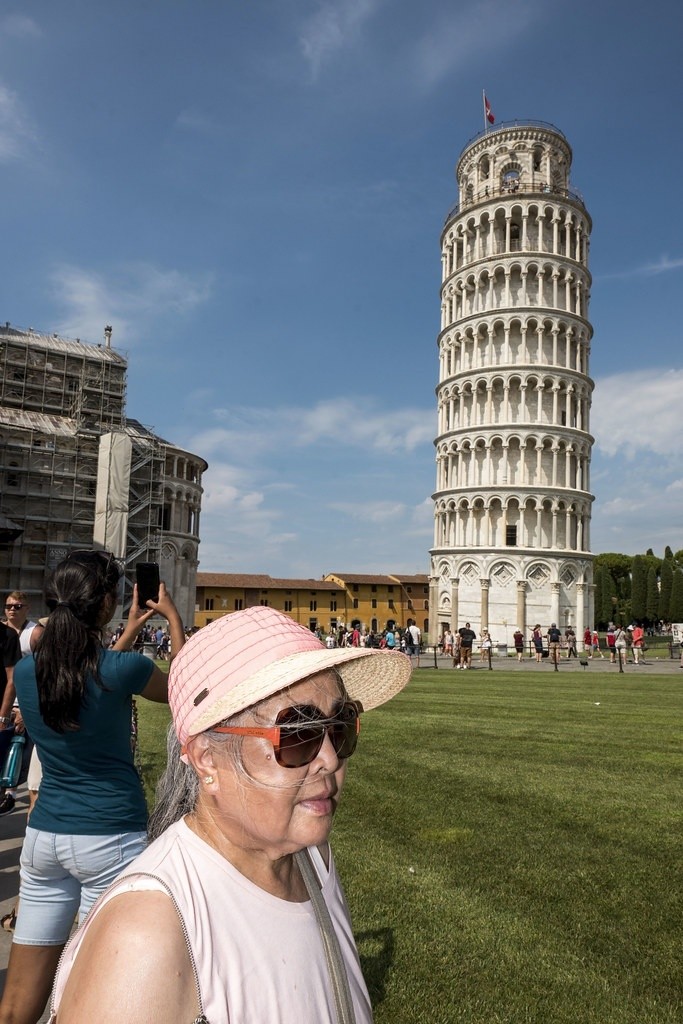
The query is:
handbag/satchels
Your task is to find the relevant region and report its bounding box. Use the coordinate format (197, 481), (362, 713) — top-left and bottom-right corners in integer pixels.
(641, 641), (648, 652)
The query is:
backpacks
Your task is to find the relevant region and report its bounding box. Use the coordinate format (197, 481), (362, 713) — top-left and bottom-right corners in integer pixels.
(404, 627), (414, 647)
(549, 628), (559, 642)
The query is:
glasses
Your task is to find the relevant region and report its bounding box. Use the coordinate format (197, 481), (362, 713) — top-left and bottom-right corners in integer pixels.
(66, 549), (115, 578)
(208, 701), (361, 768)
(4, 604), (26, 610)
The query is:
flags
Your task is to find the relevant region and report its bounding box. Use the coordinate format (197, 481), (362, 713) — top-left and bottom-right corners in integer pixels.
(485, 97), (494, 124)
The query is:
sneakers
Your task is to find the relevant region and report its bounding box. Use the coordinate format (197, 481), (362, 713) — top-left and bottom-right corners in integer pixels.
(456, 664), (464, 670)
(463, 665), (467, 670)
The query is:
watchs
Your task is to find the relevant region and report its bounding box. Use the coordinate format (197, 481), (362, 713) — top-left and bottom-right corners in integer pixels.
(0, 717), (10, 724)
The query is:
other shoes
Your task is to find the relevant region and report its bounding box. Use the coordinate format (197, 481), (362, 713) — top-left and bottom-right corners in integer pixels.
(1, 908), (17, 934)
(0, 793), (15, 816)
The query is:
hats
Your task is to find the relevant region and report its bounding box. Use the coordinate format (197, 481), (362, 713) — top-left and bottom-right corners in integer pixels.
(482, 627), (488, 631)
(167, 606), (413, 765)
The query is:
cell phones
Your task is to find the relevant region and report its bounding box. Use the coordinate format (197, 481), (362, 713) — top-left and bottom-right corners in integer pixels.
(137, 562), (159, 610)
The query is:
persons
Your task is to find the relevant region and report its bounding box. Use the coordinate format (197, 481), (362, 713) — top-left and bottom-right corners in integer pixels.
(46, 606), (413, 1024)
(440, 623), (491, 669)
(534, 624), (543, 662)
(0, 590), (47, 935)
(0, 550), (184, 1024)
(548, 623), (561, 665)
(104, 622), (201, 661)
(584, 626), (604, 658)
(314, 620), (422, 667)
(605, 621), (653, 664)
(565, 626), (579, 658)
(514, 631), (525, 662)
